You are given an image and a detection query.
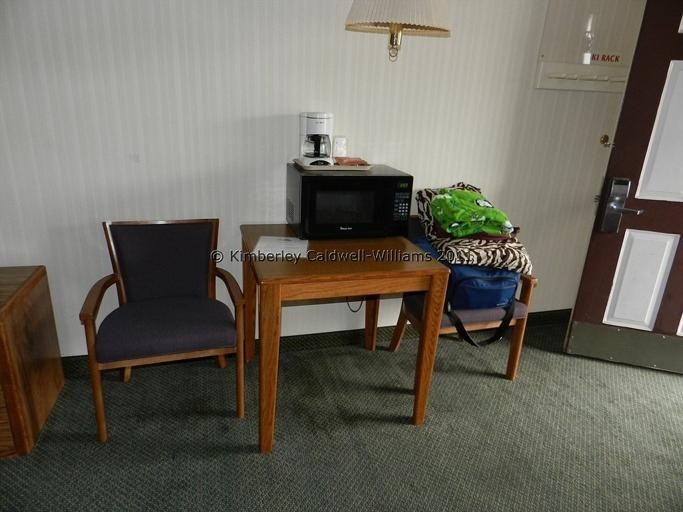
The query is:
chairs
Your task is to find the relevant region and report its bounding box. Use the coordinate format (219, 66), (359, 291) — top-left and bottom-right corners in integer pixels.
(79, 218), (248, 443)
(389, 215), (538, 394)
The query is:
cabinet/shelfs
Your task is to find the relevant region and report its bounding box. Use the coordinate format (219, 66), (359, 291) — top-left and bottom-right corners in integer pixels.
(0, 266), (65, 460)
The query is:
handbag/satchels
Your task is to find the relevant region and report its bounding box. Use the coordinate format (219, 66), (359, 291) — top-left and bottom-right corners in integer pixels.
(414, 239), (523, 310)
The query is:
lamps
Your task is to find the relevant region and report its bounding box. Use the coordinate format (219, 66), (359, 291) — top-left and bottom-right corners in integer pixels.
(345, 0), (450, 61)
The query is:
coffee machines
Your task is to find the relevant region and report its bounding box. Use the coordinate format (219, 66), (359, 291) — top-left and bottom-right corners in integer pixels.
(298, 111), (335, 166)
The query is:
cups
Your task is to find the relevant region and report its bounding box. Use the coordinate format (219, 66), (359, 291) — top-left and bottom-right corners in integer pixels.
(333, 138), (347, 157)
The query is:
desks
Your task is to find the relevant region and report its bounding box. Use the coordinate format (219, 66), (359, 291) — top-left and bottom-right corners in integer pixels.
(241, 225), (451, 454)
